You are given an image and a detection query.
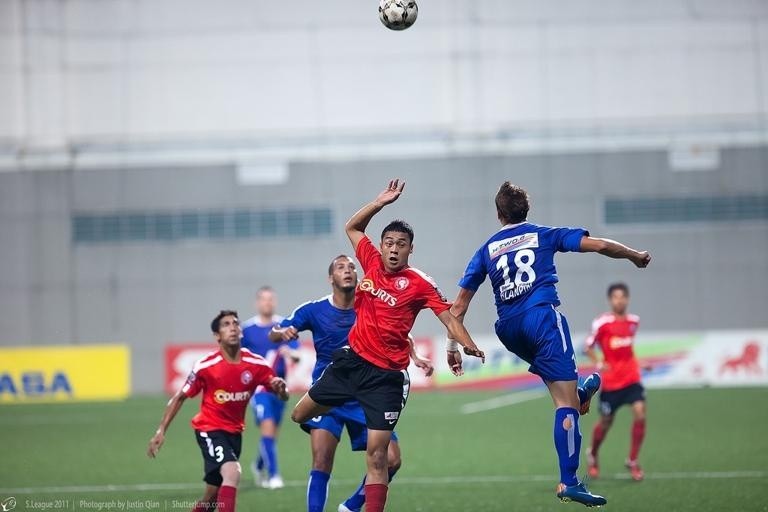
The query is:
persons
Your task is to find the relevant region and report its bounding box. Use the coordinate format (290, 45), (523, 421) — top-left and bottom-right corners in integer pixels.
(267, 254), (434, 512)
(289, 177), (486, 512)
(240, 284), (299, 490)
(582, 280), (648, 481)
(444, 179), (653, 508)
(147, 308), (291, 512)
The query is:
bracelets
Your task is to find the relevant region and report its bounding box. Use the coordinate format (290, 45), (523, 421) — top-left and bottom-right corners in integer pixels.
(446, 338), (458, 352)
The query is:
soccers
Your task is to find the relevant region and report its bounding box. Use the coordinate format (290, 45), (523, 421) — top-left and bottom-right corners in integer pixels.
(379, 0), (418, 30)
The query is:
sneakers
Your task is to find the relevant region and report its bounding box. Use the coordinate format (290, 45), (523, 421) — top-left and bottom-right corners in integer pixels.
(585, 447), (599, 478)
(250, 459), (267, 488)
(555, 480), (593, 495)
(624, 457), (644, 481)
(578, 372), (601, 415)
(262, 476), (284, 490)
